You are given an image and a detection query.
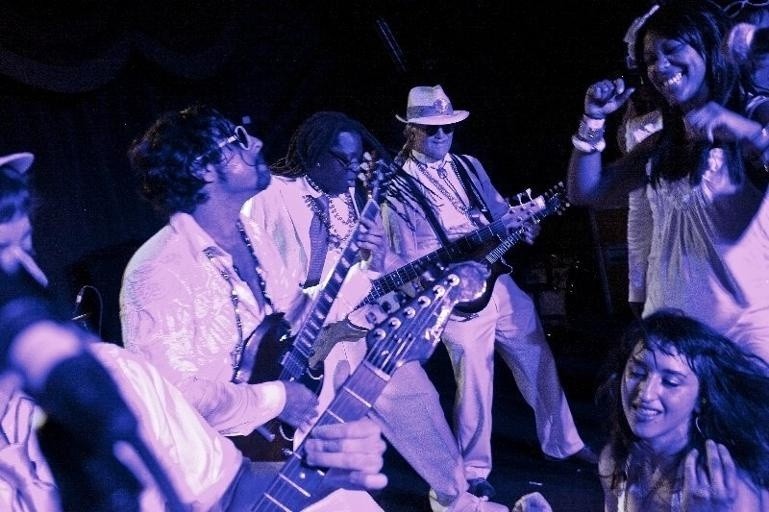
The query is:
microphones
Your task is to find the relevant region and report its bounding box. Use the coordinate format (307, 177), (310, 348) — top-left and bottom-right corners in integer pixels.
(727, 23), (769, 66)
(1, 274), (138, 440)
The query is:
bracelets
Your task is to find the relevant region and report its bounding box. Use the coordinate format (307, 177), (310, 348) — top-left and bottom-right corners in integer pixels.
(742, 125), (769, 174)
(569, 115), (608, 154)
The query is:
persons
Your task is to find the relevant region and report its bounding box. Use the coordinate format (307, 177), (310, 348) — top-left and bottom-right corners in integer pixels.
(565, 0), (769, 374)
(115, 103), (391, 512)
(380, 82), (612, 500)
(238, 110), (512, 511)
(0, 151), (390, 512)
(615, 72), (670, 322)
(591, 304), (768, 512)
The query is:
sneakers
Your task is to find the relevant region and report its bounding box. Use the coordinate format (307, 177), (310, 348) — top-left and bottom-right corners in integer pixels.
(428, 489), (510, 512)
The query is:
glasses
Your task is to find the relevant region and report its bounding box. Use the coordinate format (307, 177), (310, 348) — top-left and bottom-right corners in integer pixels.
(194, 125), (251, 161)
(330, 148), (359, 171)
(420, 123), (457, 138)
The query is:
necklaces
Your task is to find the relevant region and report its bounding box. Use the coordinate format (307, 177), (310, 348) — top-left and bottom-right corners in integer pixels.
(207, 218), (278, 375)
(408, 154), (476, 215)
(297, 172), (358, 241)
(624, 442), (698, 512)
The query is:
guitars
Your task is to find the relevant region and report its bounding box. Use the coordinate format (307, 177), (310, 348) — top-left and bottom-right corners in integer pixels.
(232, 151), (570, 512)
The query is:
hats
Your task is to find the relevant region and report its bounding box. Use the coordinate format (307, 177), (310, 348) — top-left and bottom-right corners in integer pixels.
(395, 84), (470, 126)
(0, 150), (35, 175)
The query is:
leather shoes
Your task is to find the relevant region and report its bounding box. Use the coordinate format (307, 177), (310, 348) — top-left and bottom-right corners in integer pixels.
(465, 476), (497, 499)
(543, 443), (599, 474)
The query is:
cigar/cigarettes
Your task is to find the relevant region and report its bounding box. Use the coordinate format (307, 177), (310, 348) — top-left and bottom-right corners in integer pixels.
(11, 247), (50, 288)
